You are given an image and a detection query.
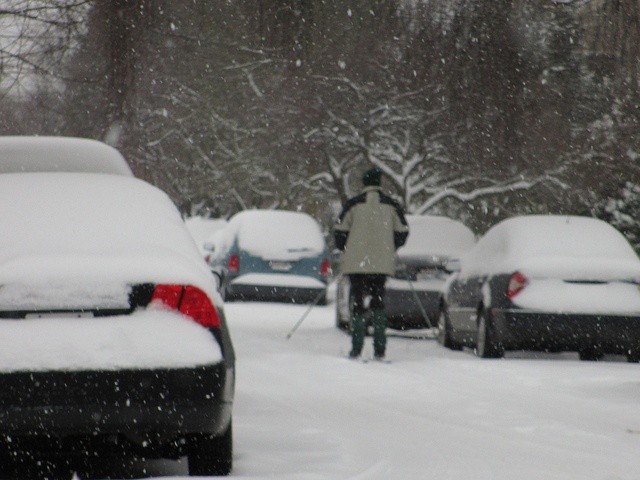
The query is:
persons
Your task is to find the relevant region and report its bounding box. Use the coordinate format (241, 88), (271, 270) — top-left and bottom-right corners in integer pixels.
(332, 166), (410, 360)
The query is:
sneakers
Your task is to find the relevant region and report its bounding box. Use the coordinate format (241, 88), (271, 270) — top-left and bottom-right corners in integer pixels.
(374, 349), (384, 358)
(350, 348), (361, 356)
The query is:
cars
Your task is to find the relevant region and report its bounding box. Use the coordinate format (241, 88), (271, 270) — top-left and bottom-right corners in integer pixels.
(435, 213), (640, 362)
(335, 214), (477, 337)
(0, 172), (235, 480)
(203, 209), (328, 307)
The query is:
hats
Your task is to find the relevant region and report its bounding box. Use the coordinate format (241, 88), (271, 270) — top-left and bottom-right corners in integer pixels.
(362, 168), (382, 185)
